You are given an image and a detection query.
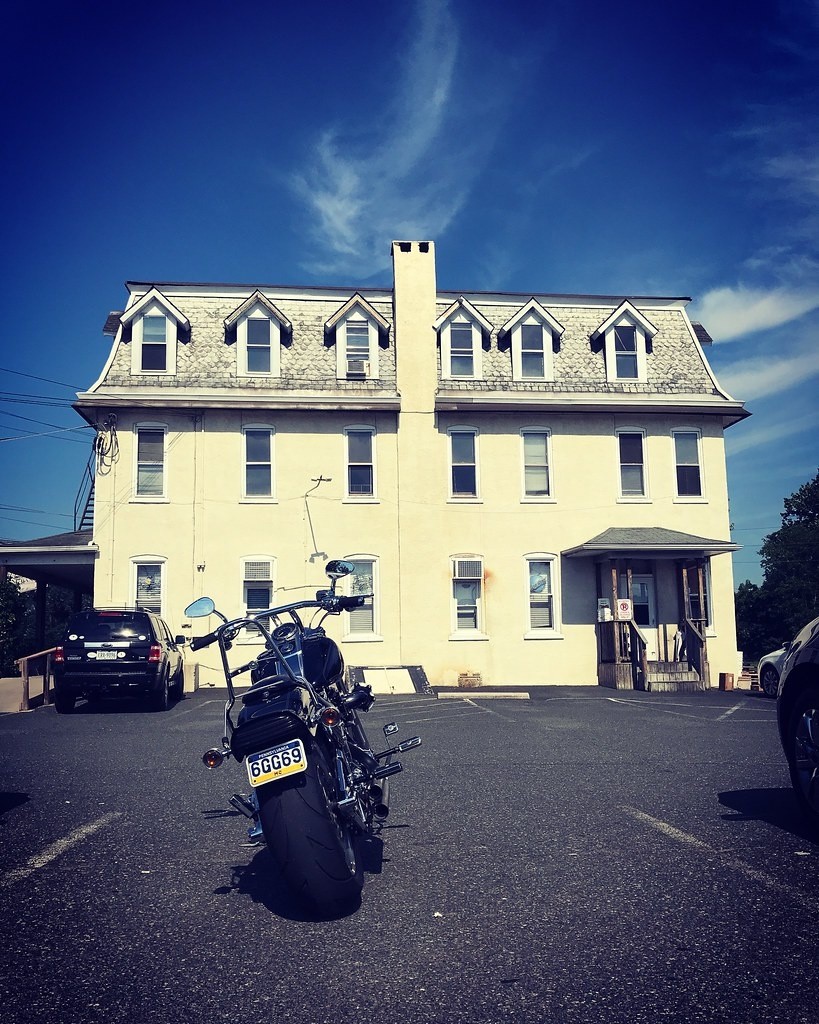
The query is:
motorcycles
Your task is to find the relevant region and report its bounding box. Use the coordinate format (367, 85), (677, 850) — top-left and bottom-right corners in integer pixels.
(182, 558), (423, 904)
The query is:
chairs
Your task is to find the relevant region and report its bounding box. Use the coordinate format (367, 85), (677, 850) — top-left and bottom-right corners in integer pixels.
(94, 625), (110, 640)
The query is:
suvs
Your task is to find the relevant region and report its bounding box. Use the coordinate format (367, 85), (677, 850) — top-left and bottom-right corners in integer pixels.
(53, 604), (186, 715)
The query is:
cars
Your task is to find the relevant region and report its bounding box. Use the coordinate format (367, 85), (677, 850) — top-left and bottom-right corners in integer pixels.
(757, 641), (791, 699)
(777, 615), (819, 834)
